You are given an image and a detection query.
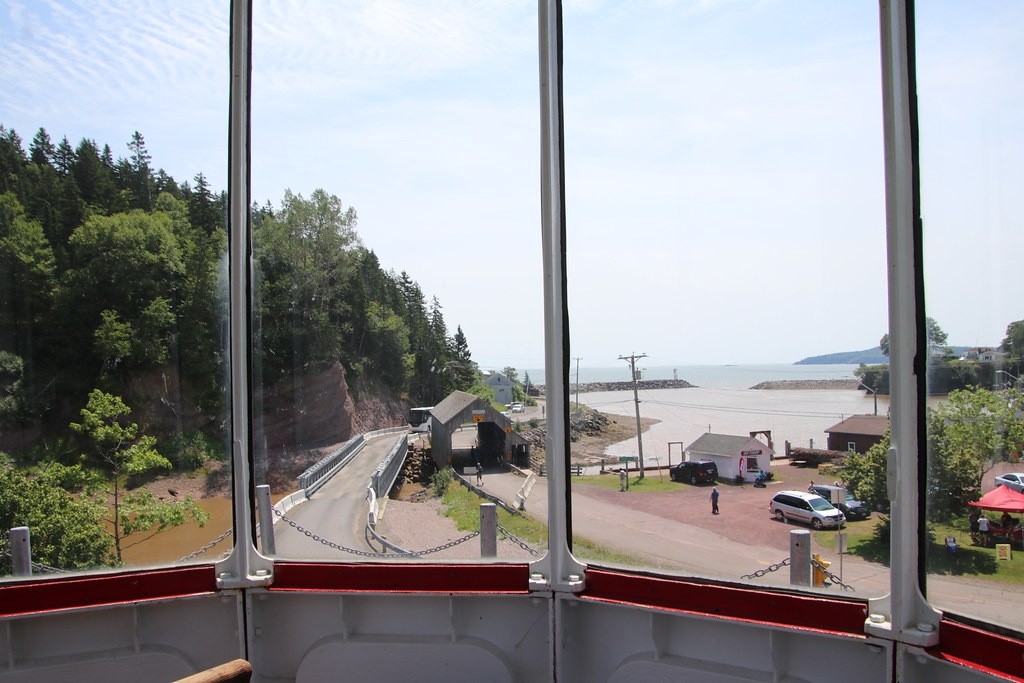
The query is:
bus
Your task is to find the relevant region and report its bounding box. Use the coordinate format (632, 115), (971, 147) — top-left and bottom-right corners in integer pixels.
(408, 407), (434, 434)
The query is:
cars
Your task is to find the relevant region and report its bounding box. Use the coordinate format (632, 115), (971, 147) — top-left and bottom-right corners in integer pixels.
(994, 473), (1024, 495)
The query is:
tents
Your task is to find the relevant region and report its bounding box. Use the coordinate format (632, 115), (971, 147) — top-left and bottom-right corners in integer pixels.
(966, 484), (1024, 542)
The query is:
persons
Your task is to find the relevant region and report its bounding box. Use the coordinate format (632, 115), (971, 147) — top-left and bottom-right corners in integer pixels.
(969, 507), (1020, 548)
(618, 468), (626, 492)
(476, 463), (484, 486)
(709, 487), (720, 515)
(470, 445), (477, 462)
(807, 480), (815, 494)
(754, 470), (766, 486)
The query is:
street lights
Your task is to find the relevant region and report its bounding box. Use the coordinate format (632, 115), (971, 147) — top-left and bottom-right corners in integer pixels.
(842, 376), (877, 415)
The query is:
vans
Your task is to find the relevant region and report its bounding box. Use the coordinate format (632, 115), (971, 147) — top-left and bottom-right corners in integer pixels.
(513, 404), (525, 413)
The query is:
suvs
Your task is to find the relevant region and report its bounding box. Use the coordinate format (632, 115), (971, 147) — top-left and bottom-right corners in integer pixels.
(809, 485), (871, 521)
(505, 402), (521, 409)
(769, 490), (846, 530)
(669, 459), (718, 485)
(501, 412), (510, 418)
